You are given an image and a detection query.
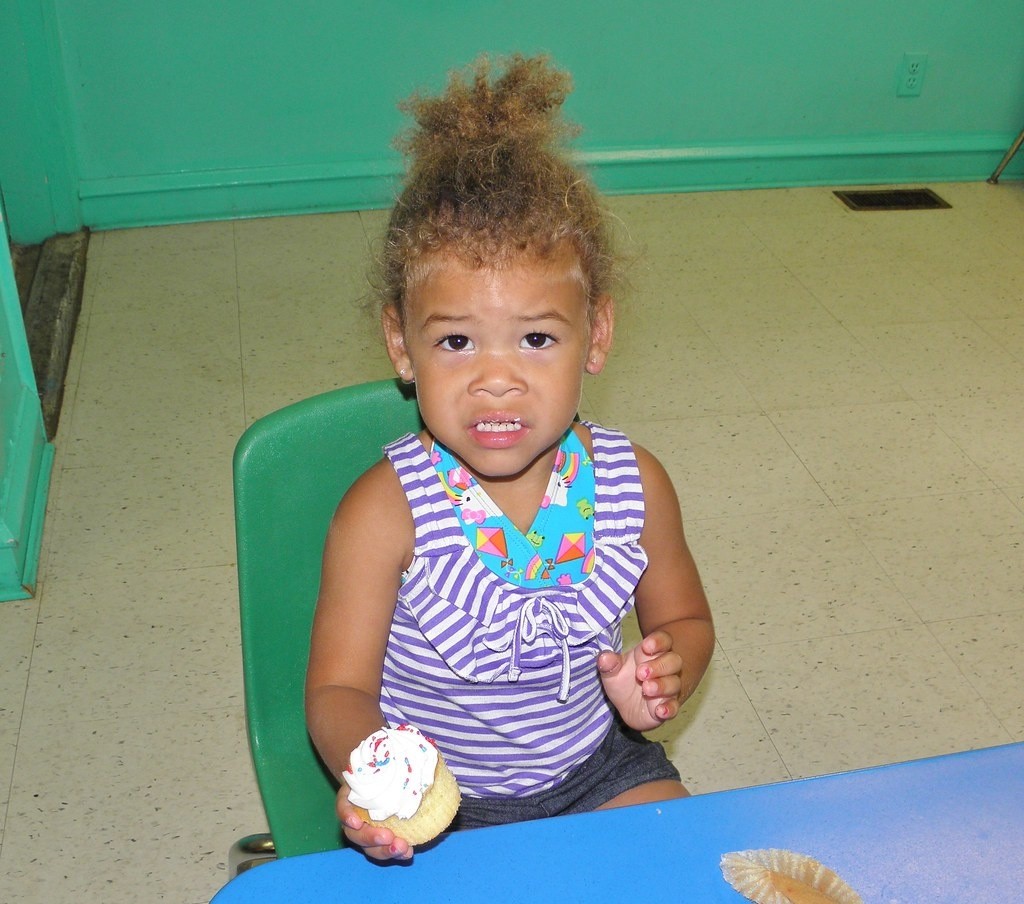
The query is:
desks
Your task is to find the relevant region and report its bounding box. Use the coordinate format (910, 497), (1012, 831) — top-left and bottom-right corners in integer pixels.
(211, 742), (1024, 904)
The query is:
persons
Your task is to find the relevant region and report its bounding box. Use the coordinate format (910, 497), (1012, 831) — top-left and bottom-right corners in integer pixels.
(306, 56), (716, 861)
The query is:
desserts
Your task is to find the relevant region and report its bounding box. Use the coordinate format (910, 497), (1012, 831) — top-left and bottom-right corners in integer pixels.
(342, 724), (461, 842)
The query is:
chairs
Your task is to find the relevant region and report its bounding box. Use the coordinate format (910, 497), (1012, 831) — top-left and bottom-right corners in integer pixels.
(229, 378), (423, 886)
